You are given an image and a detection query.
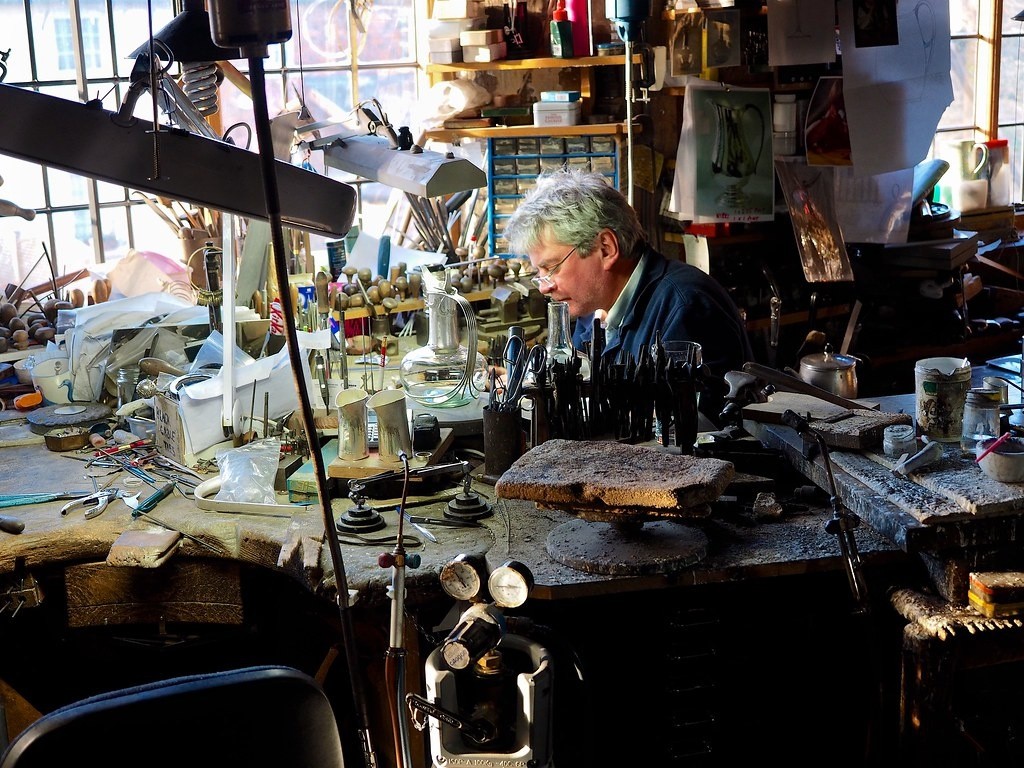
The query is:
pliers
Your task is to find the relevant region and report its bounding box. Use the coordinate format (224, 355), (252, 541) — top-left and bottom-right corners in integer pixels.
(61, 487), (119, 521)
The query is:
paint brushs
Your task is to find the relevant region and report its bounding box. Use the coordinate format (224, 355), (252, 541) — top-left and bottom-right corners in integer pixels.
(399, 193), (490, 255)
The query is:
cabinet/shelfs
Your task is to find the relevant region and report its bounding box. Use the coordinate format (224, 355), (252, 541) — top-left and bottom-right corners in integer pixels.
(487, 136), (627, 258)
(331, 0), (652, 323)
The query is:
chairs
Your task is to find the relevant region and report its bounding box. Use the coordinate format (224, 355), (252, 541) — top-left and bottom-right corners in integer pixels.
(0, 665), (344, 768)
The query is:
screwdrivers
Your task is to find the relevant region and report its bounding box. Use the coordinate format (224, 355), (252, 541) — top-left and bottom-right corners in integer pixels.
(315, 271), (332, 379)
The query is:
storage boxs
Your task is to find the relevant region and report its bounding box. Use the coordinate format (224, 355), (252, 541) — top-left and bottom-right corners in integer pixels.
(153, 357), (324, 468)
(533, 90), (583, 126)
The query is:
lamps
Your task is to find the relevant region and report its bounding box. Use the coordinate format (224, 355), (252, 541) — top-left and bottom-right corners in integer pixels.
(0, 0), (485, 239)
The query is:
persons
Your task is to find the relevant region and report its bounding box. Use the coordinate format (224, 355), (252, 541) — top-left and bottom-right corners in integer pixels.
(485, 172), (754, 440)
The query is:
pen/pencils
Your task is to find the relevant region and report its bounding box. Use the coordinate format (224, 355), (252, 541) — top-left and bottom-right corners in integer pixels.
(131, 481), (176, 518)
(395, 506), (436, 549)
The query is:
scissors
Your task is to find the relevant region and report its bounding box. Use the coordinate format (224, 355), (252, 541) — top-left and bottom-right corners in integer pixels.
(495, 337), (546, 417)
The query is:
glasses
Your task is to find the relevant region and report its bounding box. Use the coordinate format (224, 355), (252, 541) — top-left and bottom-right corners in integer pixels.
(531, 224), (621, 290)
